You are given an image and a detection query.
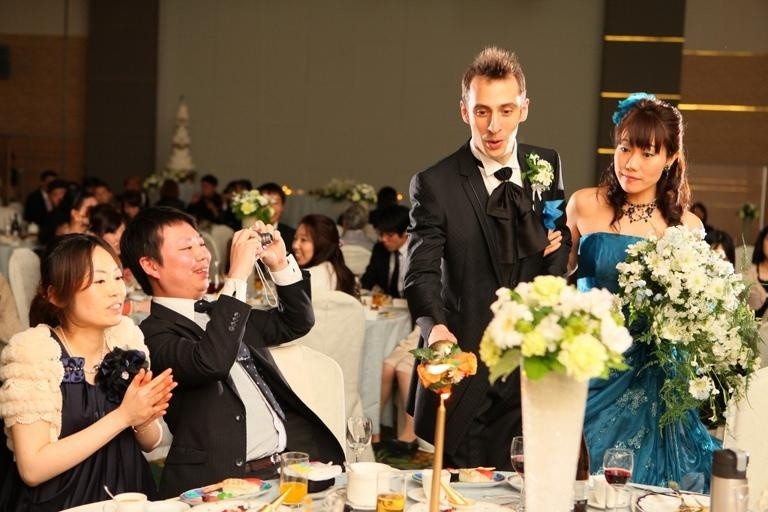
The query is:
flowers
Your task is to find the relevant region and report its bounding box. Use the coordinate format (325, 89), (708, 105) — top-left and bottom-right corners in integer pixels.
(521, 152), (554, 211)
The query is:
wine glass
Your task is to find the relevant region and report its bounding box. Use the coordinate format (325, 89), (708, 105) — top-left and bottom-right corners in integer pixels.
(603, 447), (634, 512)
(508, 435), (527, 512)
(344, 417), (374, 461)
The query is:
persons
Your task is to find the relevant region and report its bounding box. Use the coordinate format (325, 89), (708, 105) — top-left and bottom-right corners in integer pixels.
(690, 200), (715, 232)
(704, 229), (735, 269)
(0, 231), (178, 511)
(118, 203), (347, 500)
(742, 227), (768, 322)
(372, 324), (420, 457)
(542, 90), (723, 496)
(401, 43), (574, 473)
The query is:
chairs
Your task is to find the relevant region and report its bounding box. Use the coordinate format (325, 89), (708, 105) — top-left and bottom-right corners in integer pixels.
(0, 202), (768, 512)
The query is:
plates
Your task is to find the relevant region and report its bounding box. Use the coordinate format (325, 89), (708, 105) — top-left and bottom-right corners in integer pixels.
(60, 468), (710, 511)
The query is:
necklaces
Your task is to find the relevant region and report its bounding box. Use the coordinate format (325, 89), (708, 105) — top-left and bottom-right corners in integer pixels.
(621, 198), (658, 224)
(55, 325), (106, 374)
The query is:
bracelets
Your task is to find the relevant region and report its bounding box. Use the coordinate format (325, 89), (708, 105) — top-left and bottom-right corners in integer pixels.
(128, 426), (148, 435)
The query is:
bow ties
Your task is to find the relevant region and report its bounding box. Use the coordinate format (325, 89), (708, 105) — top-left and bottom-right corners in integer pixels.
(484, 167), (550, 266)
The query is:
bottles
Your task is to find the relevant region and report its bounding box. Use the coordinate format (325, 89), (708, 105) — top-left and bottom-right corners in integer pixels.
(574, 431), (589, 512)
(708, 449), (750, 510)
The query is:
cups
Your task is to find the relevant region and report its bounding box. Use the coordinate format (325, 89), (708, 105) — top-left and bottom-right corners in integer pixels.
(345, 461), (392, 504)
(280, 452), (307, 504)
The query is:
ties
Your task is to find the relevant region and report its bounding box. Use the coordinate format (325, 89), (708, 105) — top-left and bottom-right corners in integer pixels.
(194, 298), (289, 423)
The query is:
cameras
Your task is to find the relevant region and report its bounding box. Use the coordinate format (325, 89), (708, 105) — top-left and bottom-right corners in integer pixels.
(252, 231), (275, 261)
